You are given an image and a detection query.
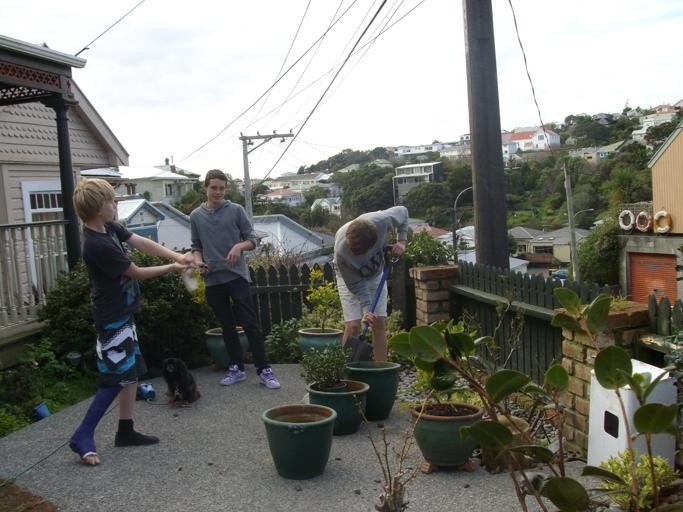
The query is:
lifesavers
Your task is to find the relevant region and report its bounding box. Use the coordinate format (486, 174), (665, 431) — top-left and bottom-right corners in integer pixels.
(636, 211), (653, 232)
(619, 209), (635, 230)
(653, 211), (672, 235)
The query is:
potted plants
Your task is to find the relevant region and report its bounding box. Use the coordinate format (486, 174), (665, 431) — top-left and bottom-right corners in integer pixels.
(191, 267), (249, 369)
(15, 374), (51, 419)
(460, 283), (530, 450)
(298, 340), (353, 393)
(411, 324), (484, 468)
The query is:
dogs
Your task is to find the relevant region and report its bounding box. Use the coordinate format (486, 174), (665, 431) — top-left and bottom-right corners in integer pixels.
(161, 356), (201, 403)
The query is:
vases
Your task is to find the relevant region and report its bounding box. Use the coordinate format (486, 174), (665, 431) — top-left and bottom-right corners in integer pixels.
(296, 269), (343, 354)
(344, 360), (402, 422)
(304, 380), (370, 436)
(261, 404), (337, 479)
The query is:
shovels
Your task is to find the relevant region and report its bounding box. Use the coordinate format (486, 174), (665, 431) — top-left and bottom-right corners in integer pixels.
(342, 246), (403, 361)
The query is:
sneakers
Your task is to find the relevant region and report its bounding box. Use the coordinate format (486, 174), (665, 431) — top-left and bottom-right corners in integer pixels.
(115, 430), (159, 446)
(220, 364), (246, 386)
(260, 368), (280, 388)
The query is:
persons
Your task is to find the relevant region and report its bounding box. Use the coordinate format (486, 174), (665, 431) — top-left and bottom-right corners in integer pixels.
(331, 205), (408, 363)
(190, 169), (279, 389)
(67, 178), (195, 466)
